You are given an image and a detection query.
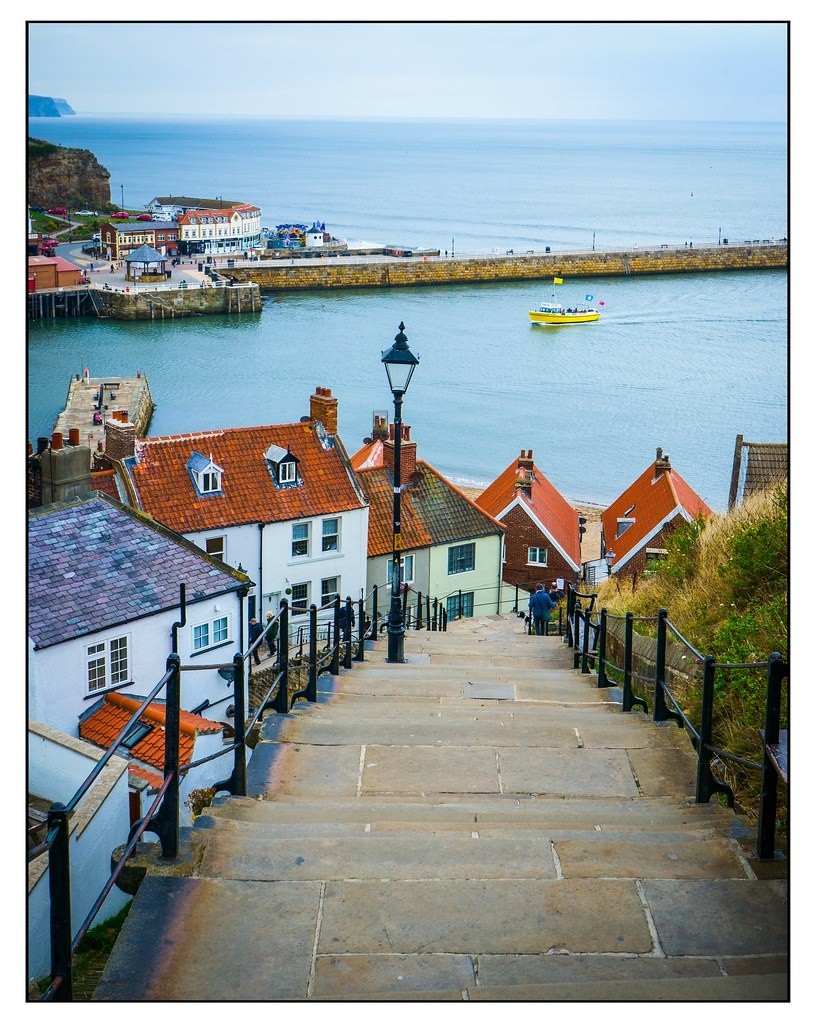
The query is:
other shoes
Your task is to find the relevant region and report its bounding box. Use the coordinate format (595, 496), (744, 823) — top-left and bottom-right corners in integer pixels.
(267, 652), (274, 657)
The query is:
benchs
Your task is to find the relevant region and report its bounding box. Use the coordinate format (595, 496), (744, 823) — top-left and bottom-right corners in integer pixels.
(104, 382), (120, 390)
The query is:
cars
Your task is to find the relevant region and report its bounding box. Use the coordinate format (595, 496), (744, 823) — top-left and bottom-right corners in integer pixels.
(28, 203), (42, 213)
(92, 233), (101, 242)
(48, 207), (67, 215)
(74, 210), (98, 217)
(136, 215), (152, 221)
(43, 240), (59, 247)
(111, 212), (129, 219)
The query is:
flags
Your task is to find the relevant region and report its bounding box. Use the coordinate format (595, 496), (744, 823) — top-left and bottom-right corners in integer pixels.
(598, 300), (605, 306)
(554, 278), (563, 284)
(585, 295), (593, 301)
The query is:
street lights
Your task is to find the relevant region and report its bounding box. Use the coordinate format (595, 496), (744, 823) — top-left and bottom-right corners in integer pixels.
(718, 227), (722, 245)
(380, 335), (419, 664)
(216, 196), (222, 209)
(592, 231), (595, 250)
(80, 356), (84, 383)
(452, 236), (454, 257)
(121, 184), (124, 213)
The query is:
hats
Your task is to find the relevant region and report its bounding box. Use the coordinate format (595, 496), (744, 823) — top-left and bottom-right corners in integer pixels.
(250, 619), (256, 623)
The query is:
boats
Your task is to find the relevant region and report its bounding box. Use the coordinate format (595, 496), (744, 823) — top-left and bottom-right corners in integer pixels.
(528, 276), (600, 324)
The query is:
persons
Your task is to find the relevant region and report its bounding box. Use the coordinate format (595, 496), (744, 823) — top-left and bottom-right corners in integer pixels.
(249, 618), (263, 665)
(179, 279), (188, 288)
(172, 258), (177, 267)
(109, 264), (114, 273)
(265, 611), (279, 656)
(84, 269), (87, 276)
(153, 268), (157, 273)
(529, 583), (558, 635)
(91, 263), (94, 270)
(549, 582), (564, 602)
(690, 242), (692, 247)
(685, 242), (687, 248)
(340, 602), (355, 642)
(95, 412), (102, 424)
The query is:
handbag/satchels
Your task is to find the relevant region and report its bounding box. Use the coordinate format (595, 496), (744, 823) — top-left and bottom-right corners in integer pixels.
(542, 611), (552, 622)
